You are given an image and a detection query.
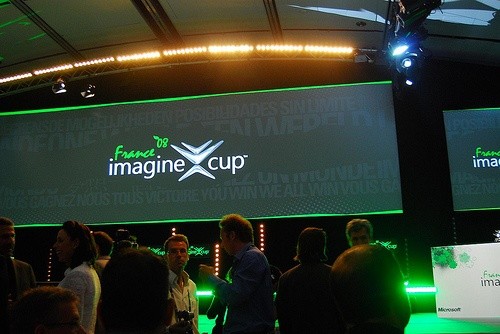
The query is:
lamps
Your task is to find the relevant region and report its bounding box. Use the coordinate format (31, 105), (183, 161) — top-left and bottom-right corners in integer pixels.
(53, 75), (68, 94)
(80, 77), (97, 98)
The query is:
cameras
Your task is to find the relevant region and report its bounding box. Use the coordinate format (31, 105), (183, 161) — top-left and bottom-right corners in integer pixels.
(177, 310), (194, 331)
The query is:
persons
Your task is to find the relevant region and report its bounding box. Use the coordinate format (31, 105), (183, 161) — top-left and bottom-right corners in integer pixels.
(0, 216), (36, 334)
(163, 234), (200, 334)
(97, 244), (174, 334)
(276, 228), (349, 334)
(89, 230), (114, 277)
(332, 243), (412, 334)
(11, 285), (87, 334)
(345, 219), (374, 246)
(54, 220), (102, 334)
(198, 214), (275, 334)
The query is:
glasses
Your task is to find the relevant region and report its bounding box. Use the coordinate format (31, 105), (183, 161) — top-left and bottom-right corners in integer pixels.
(47, 320), (84, 328)
